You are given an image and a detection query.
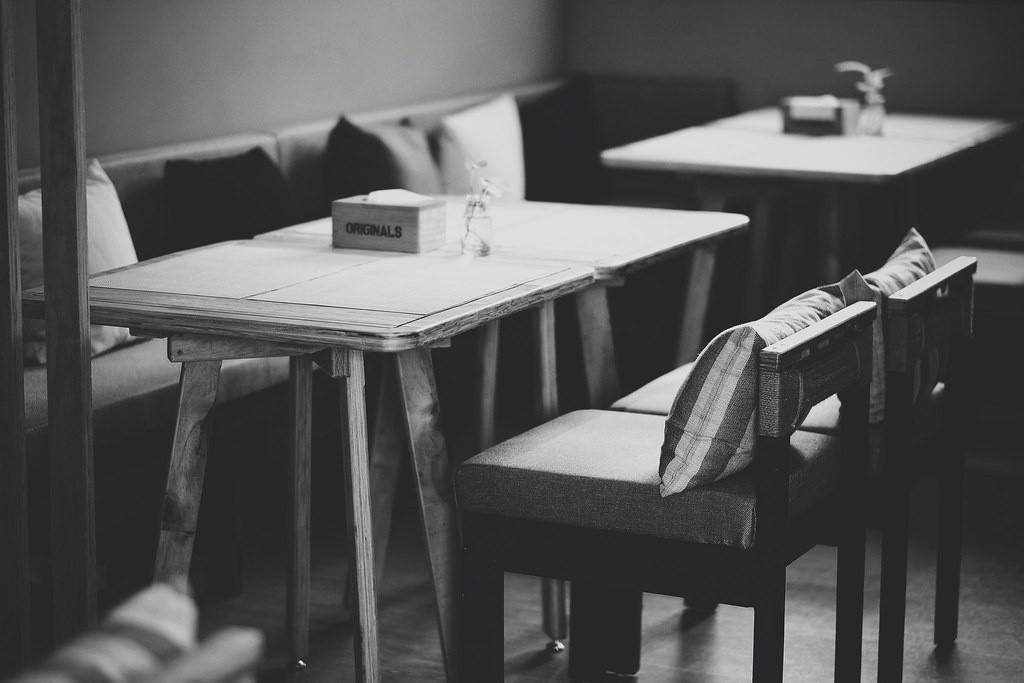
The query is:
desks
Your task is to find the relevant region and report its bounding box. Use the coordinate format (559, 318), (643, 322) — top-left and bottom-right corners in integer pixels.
(20, 189), (753, 683)
(599, 93), (1017, 368)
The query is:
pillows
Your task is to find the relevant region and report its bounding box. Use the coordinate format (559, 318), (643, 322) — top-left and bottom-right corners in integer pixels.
(658, 288), (845, 498)
(319, 115), (440, 216)
(163, 147), (301, 250)
(440, 91), (526, 201)
(19, 157), (153, 370)
(835, 225), (938, 426)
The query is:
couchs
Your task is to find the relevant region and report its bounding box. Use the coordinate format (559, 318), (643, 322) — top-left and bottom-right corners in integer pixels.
(17, 131), (337, 662)
(279, 72), (745, 374)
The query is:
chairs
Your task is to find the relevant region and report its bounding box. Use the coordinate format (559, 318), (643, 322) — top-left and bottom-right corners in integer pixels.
(455, 299), (879, 682)
(608, 257), (979, 683)
(930, 243), (1024, 289)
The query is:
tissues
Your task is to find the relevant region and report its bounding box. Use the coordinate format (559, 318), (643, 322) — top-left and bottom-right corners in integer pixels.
(332, 189), (446, 253)
(779, 94), (860, 136)
